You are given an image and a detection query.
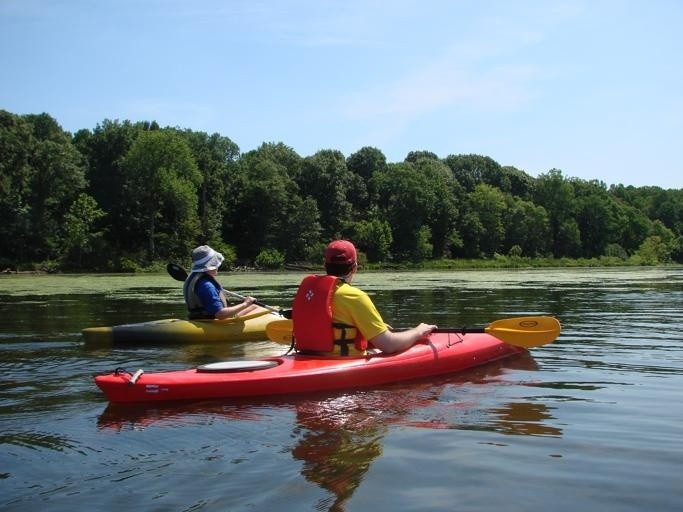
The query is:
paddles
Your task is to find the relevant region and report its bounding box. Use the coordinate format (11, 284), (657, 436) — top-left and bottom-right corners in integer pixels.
(266, 313), (562, 348)
(165, 261), (294, 320)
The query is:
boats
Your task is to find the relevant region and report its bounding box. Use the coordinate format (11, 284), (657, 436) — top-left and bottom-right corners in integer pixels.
(72, 298), (290, 346)
(91, 328), (530, 406)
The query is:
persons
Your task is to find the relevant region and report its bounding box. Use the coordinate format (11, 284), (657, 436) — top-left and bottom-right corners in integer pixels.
(292, 240), (439, 358)
(183, 245), (258, 320)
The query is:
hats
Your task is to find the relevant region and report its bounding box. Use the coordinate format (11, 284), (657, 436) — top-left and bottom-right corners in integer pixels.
(190, 245), (224, 273)
(325, 239), (358, 266)
